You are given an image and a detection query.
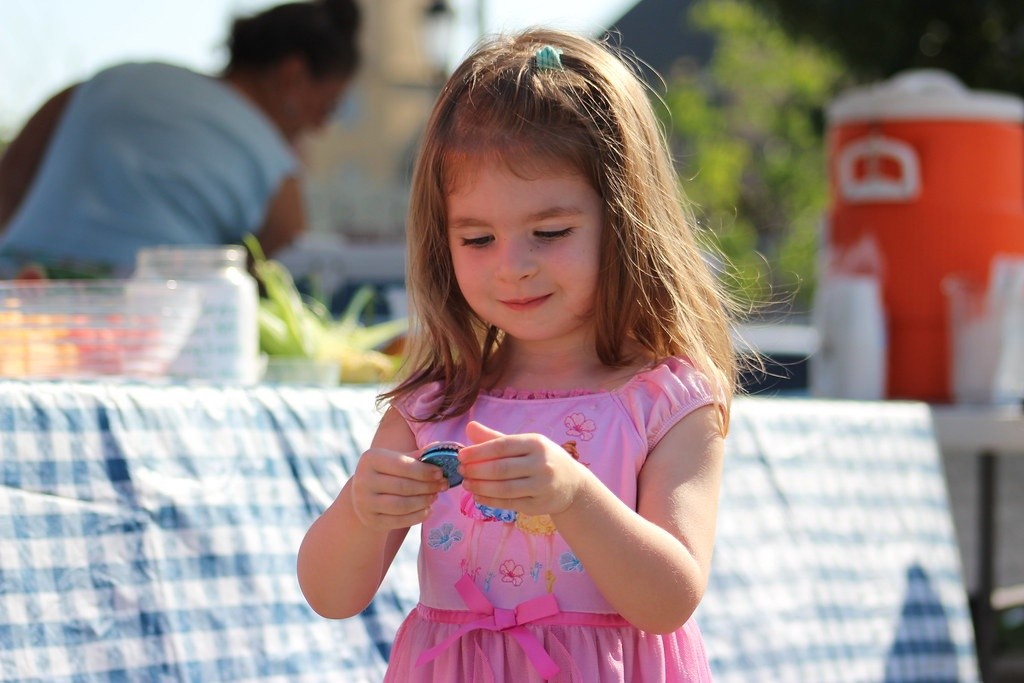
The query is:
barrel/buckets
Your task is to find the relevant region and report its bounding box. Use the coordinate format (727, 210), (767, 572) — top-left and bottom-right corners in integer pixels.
(824, 72), (1024, 400)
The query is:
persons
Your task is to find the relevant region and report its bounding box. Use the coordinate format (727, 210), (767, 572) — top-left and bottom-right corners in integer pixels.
(297, 30), (734, 683)
(0, 0), (365, 282)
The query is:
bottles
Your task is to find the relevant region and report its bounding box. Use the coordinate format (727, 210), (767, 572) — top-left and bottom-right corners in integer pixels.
(130, 246), (260, 385)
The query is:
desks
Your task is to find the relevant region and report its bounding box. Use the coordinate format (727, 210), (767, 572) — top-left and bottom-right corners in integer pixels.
(0, 382), (1024, 683)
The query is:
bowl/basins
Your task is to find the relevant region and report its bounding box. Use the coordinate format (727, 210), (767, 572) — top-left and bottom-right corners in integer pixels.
(0, 281), (199, 380)
